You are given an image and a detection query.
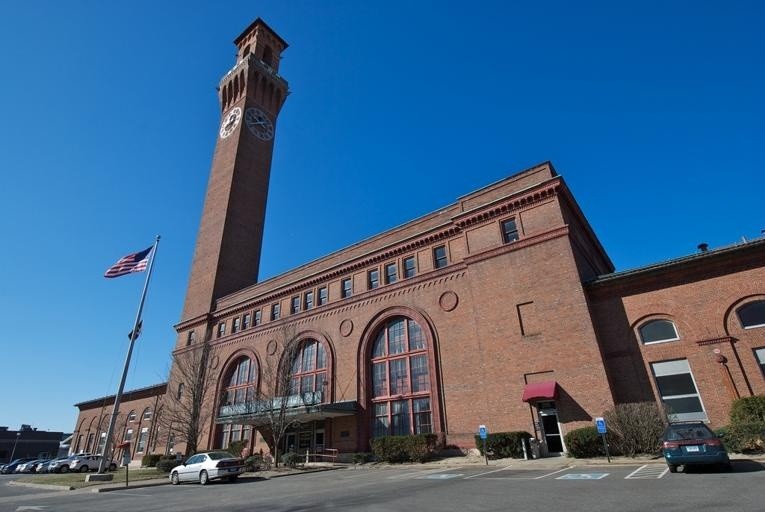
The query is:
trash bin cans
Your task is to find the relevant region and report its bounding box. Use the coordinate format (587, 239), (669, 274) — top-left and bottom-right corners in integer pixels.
(529, 437), (541, 459)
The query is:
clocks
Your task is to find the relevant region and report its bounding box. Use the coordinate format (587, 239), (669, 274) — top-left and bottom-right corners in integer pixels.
(244, 106), (275, 142)
(218, 106), (242, 139)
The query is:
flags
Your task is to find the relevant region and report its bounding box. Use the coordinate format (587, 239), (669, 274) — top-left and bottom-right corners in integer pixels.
(104, 243), (154, 279)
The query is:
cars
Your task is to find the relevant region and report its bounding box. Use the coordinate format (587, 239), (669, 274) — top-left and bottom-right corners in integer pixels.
(663, 420), (730, 473)
(0, 454), (117, 473)
(170, 451), (243, 484)
